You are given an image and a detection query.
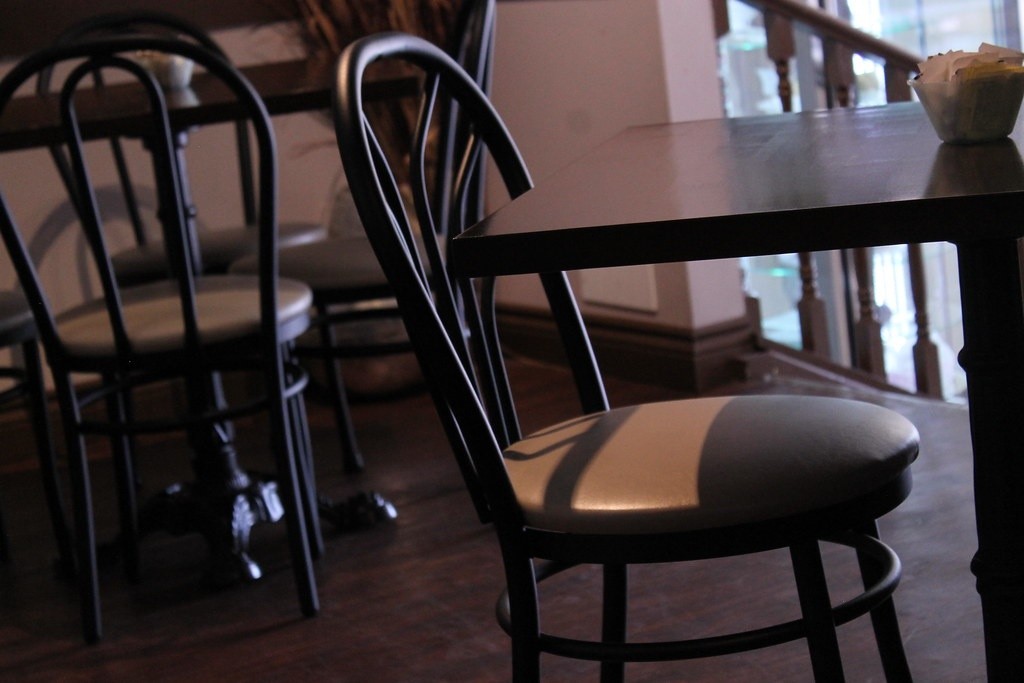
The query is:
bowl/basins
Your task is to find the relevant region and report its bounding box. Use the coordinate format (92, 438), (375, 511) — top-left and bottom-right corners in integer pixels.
(907, 72), (1024, 143)
(137, 55), (194, 90)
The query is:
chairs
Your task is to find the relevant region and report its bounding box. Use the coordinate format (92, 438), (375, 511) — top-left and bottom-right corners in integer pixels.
(0, 0), (921, 683)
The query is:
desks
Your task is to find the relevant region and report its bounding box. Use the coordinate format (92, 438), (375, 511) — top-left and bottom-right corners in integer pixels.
(449, 90), (1024, 683)
(0, 56), (423, 590)
